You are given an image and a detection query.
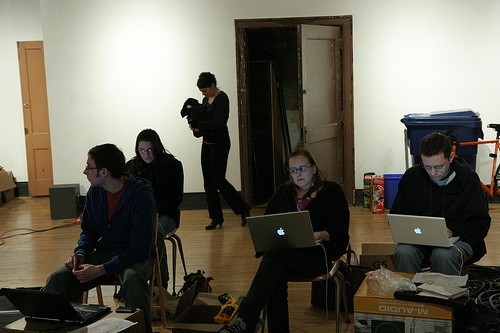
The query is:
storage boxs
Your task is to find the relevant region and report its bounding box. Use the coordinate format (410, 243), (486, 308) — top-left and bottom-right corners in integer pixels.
(358, 242), (398, 272)
(353, 272), (455, 333)
(0, 167), (16, 206)
(164, 304), (225, 333)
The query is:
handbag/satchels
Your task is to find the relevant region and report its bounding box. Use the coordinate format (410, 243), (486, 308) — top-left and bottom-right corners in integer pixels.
(150, 285), (178, 322)
(311, 250), (379, 312)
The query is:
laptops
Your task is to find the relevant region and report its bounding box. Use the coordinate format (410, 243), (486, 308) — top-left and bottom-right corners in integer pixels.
(0, 287), (111, 325)
(386, 214), (459, 248)
(245, 210), (324, 253)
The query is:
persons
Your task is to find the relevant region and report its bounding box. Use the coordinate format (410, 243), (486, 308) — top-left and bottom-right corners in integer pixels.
(190, 73), (251, 231)
(386, 131), (491, 278)
(219, 150), (350, 333)
(39, 129), (184, 333)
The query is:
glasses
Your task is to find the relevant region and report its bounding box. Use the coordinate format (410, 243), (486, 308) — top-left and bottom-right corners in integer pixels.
(286, 165), (313, 173)
(139, 148), (153, 153)
(420, 163), (444, 170)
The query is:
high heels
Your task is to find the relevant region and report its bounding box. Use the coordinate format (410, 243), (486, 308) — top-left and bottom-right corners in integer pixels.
(205, 217), (224, 230)
(241, 203), (252, 226)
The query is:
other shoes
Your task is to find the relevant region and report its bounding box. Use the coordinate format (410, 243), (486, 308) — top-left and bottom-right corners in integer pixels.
(219, 317), (256, 333)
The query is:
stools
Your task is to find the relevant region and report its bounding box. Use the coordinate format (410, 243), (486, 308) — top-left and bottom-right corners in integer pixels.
(163, 228), (188, 294)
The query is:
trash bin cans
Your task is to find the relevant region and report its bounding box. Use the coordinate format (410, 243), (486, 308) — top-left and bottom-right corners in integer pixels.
(401, 110), (484, 171)
(383, 174), (403, 209)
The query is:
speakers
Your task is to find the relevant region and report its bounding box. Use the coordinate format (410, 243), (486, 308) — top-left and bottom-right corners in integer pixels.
(49, 184), (79, 218)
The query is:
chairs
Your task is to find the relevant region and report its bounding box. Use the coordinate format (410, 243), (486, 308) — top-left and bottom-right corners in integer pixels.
(261, 232), (351, 333)
(82, 212), (167, 327)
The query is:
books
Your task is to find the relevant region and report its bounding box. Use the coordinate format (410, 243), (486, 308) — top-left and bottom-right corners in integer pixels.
(418, 281), (468, 299)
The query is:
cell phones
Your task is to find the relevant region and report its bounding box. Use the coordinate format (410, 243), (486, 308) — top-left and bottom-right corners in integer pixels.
(115, 306), (136, 312)
